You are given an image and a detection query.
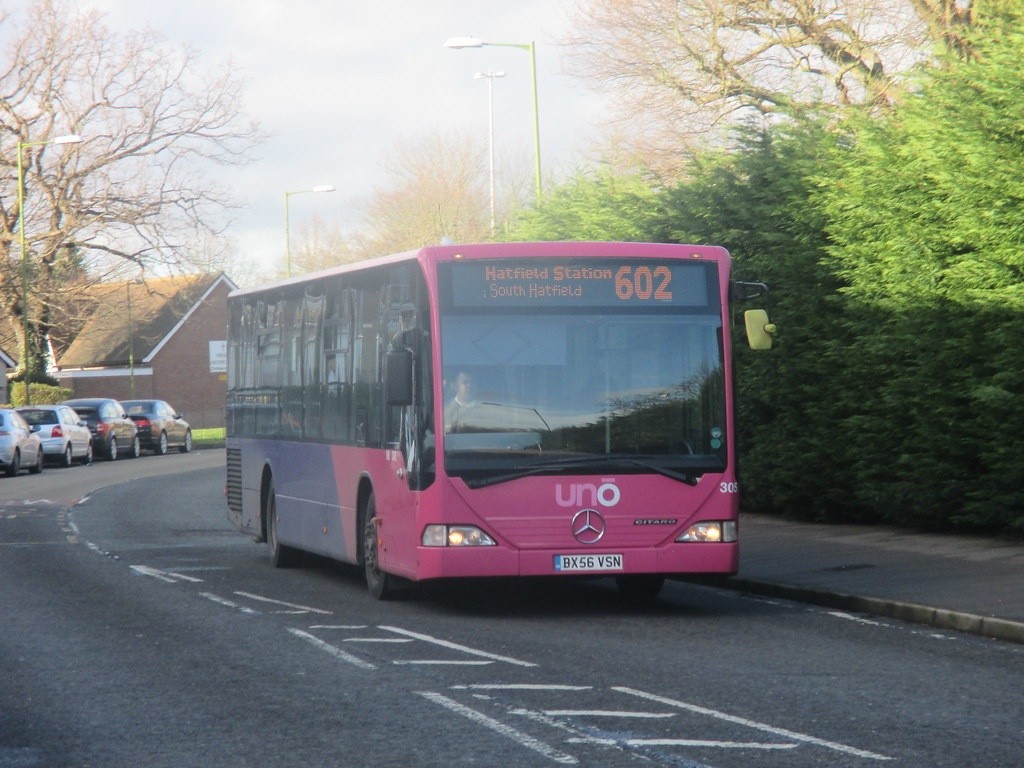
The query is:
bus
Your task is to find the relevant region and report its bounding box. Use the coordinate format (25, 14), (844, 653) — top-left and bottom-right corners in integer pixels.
(226, 242), (777, 610)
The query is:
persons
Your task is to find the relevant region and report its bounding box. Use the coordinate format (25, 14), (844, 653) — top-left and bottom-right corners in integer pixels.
(443, 369), (491, 429)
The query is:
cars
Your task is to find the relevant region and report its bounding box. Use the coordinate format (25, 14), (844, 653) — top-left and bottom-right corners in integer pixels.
(0, 407), (45, 477)
(60, 397), (142, 461)
(13, 404), (95, 468)
(117, 400), (193, 455)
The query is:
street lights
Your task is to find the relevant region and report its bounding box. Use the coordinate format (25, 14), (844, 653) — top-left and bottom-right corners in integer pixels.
(283, 184), (337, 279)
(16, 133), (82, 406)
(445, 32), (543, 211)
(126, 279), (144, 400)
